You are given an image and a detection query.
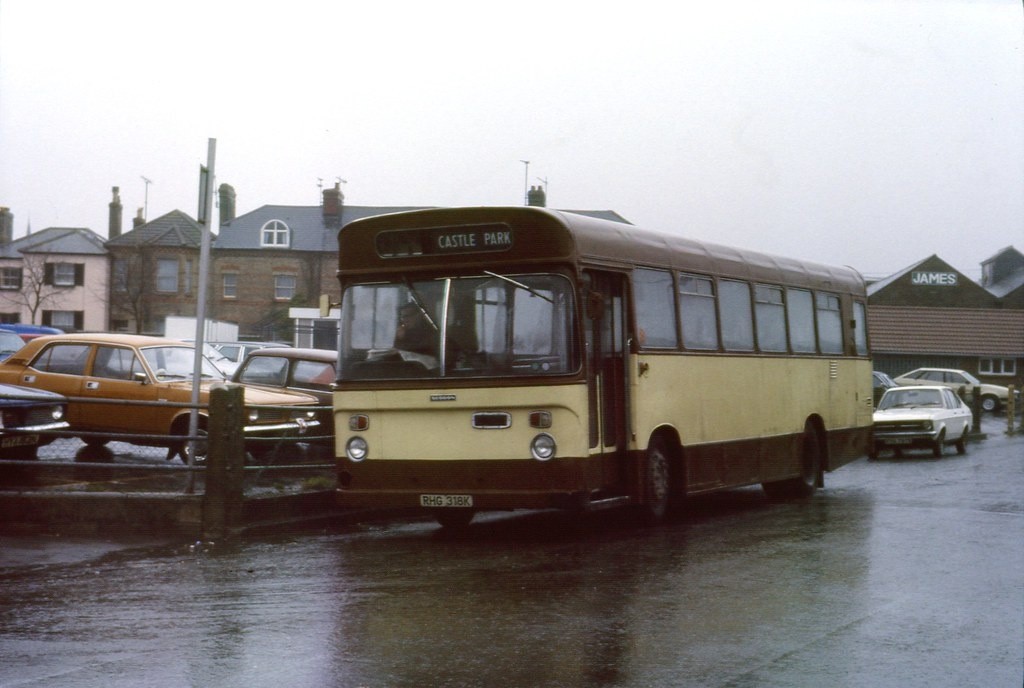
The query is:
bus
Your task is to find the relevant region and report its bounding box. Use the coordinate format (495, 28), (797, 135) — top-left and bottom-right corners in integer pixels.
(318, 205), (877, 529)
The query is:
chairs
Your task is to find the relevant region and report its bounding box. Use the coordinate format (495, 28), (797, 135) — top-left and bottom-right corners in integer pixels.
(443, 325), (479, 361)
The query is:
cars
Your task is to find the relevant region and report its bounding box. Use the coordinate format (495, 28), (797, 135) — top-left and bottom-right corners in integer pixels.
(0, 333), (321, 466)
(868, 386), (973, 459)
(873, 370), (901, 406)
(233, 349), (340, 450)
(182, 339), (292, 365)
(1, 324), (67, 345)
(0, 382), (71, 481)
(894, 367), (1020, 412)
